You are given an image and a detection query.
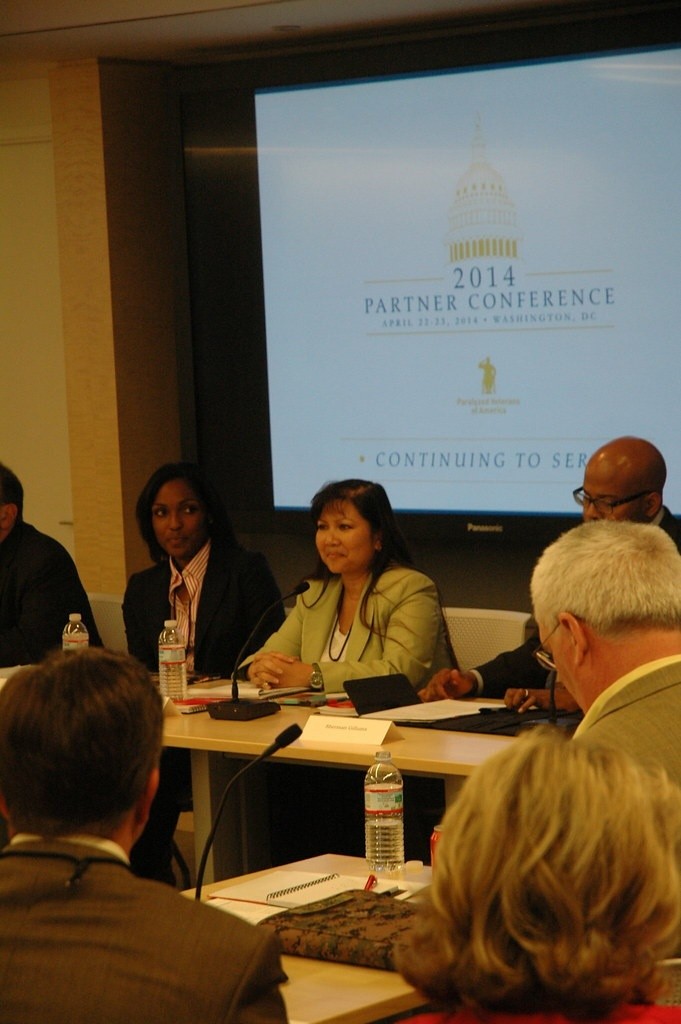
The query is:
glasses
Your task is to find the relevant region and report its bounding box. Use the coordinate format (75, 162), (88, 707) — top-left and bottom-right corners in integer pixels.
(533, 620), (560, 672)
(573, 487), (658, 515)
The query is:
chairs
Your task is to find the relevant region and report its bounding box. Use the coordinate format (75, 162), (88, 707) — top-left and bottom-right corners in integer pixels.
(444, 605), (531, 673)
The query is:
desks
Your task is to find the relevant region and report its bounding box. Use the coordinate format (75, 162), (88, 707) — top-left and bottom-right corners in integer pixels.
(182, 850), (444, 1024)
(142, 679), (558, 888)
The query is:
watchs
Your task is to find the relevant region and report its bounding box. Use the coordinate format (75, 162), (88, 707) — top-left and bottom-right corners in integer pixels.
(308, 663), (324, 692)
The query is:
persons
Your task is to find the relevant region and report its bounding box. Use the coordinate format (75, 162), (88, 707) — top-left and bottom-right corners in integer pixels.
(232, 479), (453, 691)
(390, 736), (681, 1023)
(0, 463), (104, 669)
(418, 435), (681, 714)
(531, 521), (681, 791)
(0, 649), (291, 1023)
(121, 463), (286, 680)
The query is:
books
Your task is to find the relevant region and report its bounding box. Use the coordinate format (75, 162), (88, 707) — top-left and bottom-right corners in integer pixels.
(203, 869), (416, 971)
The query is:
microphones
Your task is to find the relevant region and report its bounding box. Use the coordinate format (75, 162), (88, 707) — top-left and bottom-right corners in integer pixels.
(196, 723), (302, 901)
(205, 581), (310, 722)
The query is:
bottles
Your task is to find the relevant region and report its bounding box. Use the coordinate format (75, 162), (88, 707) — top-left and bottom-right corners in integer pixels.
(62, 614), (89, 649)
(364, 752), (404, 880)
(158, 620), (187, 704)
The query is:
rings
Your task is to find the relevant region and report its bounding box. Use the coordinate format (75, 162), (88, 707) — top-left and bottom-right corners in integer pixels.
(525, 689), (529, 697)
